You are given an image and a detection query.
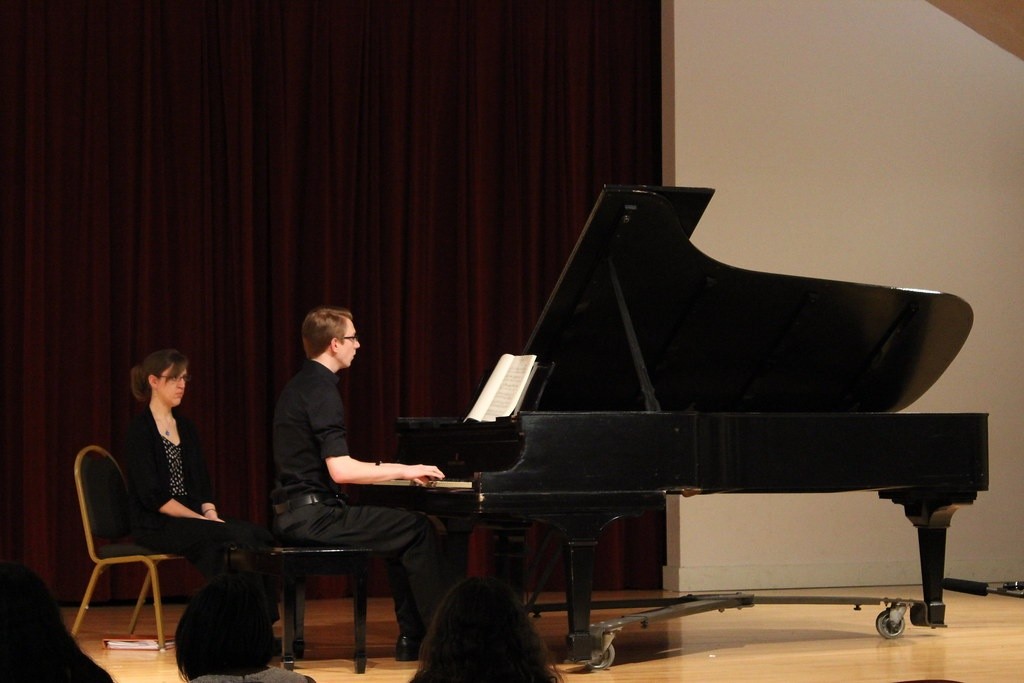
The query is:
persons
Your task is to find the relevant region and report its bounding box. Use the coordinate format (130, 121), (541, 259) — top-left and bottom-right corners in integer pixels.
(173, 580), (319, 682)
(0, 565), (116, 683)
(267, 307), (444, 664)
(118, 347), (286, 659)
(410, 574), (564, 683)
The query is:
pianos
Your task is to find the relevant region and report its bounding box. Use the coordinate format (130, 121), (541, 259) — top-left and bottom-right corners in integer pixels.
(362, 184), (992, 669)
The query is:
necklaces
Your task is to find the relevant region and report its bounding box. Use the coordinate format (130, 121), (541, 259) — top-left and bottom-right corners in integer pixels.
(146, 401), (174, 435)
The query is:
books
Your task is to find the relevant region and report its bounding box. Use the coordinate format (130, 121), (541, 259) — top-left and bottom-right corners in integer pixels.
(463, 353), (537, 421)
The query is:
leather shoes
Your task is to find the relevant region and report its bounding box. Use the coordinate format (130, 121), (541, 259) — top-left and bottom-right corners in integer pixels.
(395, 634), (423, 661)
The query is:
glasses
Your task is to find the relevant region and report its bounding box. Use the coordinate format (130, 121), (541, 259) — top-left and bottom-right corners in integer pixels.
(157, 374), (192, 382)
(344, 332), (361, 341)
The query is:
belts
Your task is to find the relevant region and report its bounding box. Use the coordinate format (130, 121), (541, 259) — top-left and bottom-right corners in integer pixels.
(274, 493), (322, 515)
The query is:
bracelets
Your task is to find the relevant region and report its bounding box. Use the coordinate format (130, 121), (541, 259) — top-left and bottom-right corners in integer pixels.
(375, 460), (382, 466)
